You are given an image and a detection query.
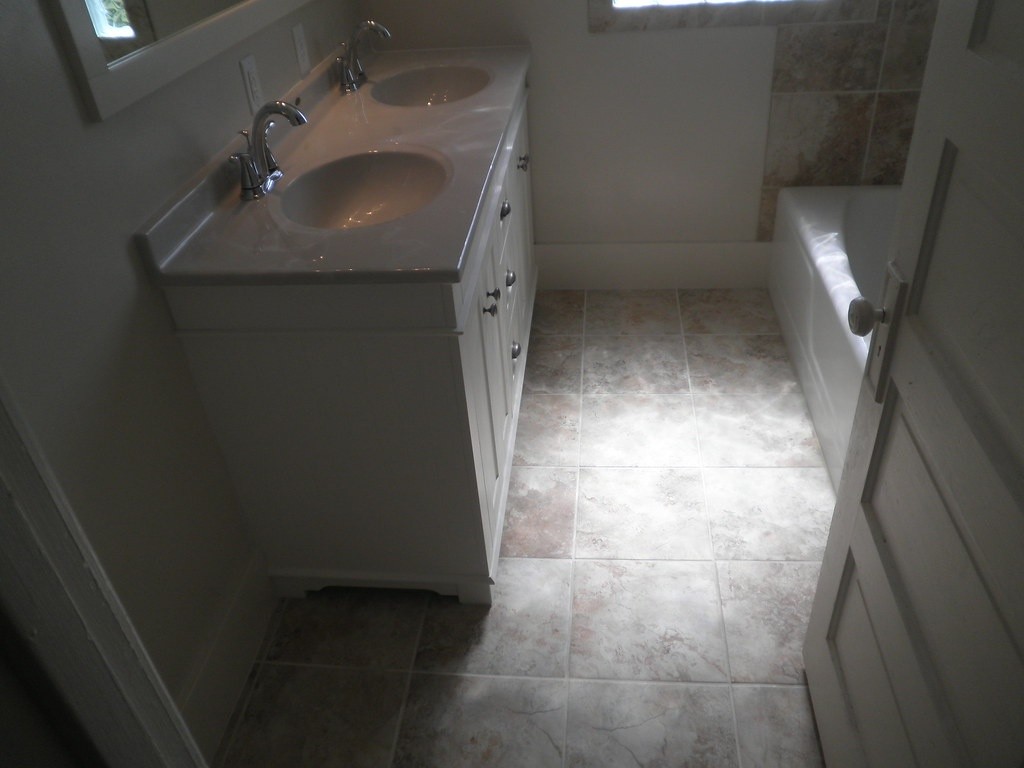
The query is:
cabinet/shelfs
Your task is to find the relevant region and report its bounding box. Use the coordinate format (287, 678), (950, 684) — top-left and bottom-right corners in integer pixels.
(159, 76), (539, 605)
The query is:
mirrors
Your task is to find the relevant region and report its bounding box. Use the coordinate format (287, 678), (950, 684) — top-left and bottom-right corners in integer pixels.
(53, 0), (308, 124)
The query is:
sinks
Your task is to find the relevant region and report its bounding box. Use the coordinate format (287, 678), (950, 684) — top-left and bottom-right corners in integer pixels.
(278, 150), (447, 229)
(370, 66), (489, 107)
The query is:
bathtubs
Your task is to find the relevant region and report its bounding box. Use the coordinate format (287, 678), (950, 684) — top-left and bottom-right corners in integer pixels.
(765, 183), (907, 498)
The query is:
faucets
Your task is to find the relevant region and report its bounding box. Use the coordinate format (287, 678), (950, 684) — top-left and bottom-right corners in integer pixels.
(228, 99), (309, 202)
(335, 18), (392, 94)
